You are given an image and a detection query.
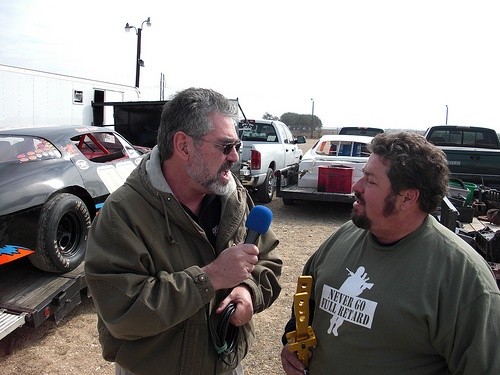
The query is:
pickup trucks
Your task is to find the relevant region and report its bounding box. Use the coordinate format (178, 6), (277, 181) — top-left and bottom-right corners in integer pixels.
(421, 125), (500, 182)
(230, 119), (307, 203)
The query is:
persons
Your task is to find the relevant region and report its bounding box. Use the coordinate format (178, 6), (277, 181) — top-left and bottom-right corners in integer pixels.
(83, 87), (283, 375)
(281, 130), (500, 375)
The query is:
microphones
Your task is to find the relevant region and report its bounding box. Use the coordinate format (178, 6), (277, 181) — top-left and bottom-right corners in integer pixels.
(244, 205), (273, 244)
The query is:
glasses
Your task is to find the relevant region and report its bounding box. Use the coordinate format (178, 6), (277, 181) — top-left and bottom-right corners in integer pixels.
(189, 135), (242, 155)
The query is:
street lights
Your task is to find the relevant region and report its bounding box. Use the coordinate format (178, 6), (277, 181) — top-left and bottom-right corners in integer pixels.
(124, 17), (152, 87)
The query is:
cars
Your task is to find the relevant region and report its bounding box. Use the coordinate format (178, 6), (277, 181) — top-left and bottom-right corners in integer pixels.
(0, 125), (147, 273)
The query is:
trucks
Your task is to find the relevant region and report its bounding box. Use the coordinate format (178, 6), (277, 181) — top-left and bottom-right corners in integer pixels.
(274, 126), (386, 205)
